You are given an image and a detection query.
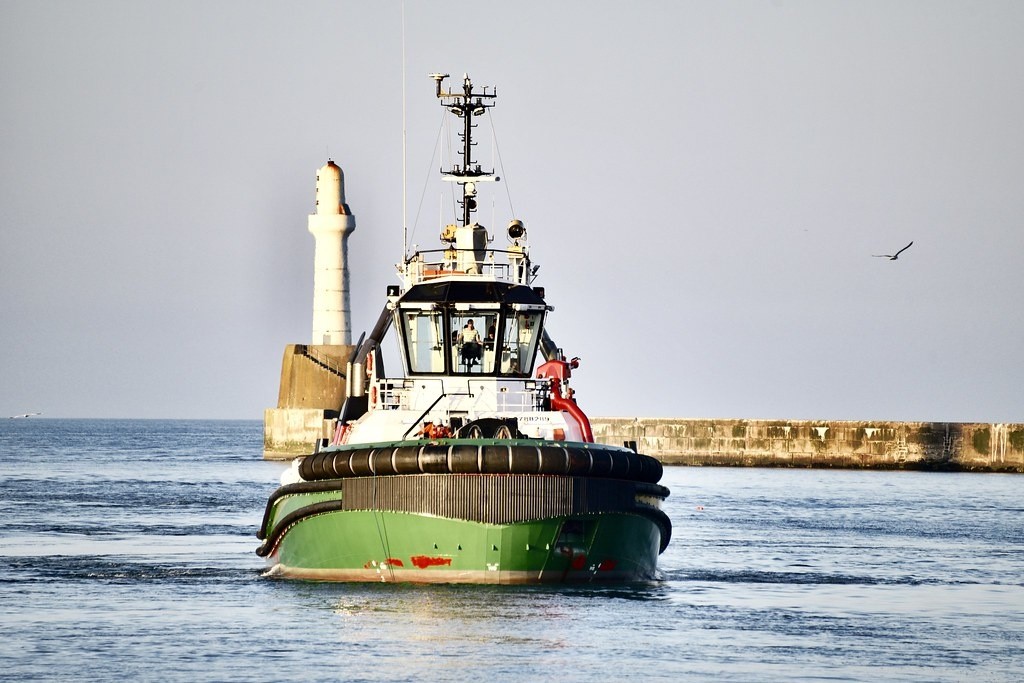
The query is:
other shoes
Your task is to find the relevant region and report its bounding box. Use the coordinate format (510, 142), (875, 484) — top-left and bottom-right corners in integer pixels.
(472, 361), (478, 365)
(462, 361), (465, 364)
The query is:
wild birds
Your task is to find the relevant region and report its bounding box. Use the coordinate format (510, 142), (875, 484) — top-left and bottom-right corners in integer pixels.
(872, 241), (913, 261)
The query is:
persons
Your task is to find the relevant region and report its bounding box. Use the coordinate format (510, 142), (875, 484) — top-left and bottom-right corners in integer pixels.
(519, 253), (530, 283)
(488, 319), (496, 351)
(458, 319), (483, 365)
(413, 418), (453, 439)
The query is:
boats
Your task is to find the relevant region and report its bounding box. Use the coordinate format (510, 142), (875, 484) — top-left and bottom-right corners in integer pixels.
(256, 73), (672, 592)
